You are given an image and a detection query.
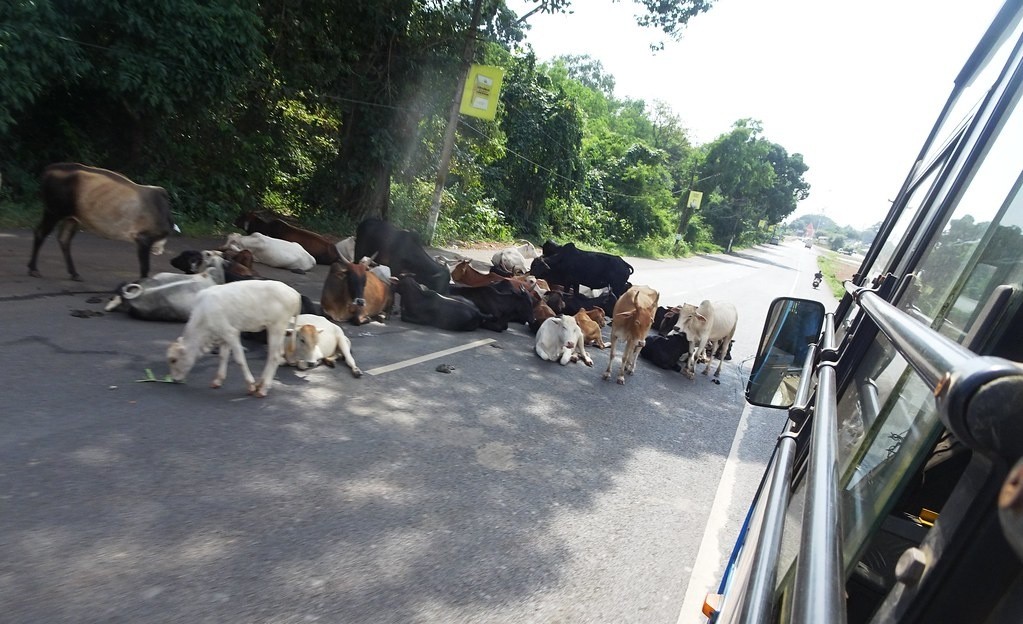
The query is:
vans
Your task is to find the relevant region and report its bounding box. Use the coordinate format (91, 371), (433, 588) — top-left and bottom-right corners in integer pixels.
(771, 236), (779, 245)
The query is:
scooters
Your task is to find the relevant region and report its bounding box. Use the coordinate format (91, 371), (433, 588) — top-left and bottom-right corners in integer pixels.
(812, 275), (822, 289)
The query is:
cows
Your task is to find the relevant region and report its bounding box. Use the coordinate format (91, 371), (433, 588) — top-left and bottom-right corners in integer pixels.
(27, 162), (182, 283)
(104, 210), (738, 397)
